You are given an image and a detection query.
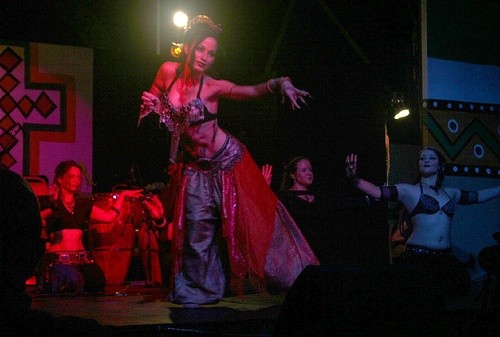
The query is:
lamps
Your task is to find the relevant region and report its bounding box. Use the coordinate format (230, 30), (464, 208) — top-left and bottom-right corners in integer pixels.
(389, 89), (412, 120)
(170, 42), (183, 57)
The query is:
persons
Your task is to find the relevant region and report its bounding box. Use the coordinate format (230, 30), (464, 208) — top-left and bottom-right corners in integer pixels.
(261, 157), (389, 260)
(346, 147), (500, 309)
(1, 151), (174, 309)
(142, 17), (320, 308)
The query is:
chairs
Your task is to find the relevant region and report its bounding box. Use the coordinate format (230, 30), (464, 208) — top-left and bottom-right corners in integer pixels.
(25, 176), (49, 209)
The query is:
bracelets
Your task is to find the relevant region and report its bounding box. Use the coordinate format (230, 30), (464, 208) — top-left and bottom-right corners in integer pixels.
(267, 77), (290, 95)
